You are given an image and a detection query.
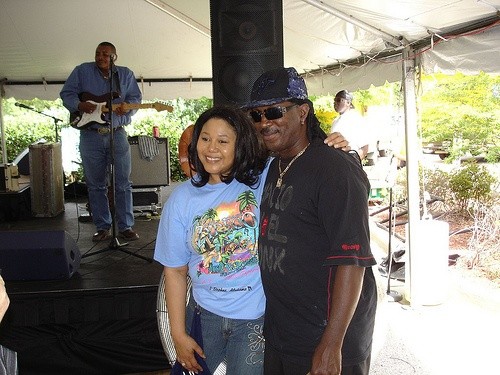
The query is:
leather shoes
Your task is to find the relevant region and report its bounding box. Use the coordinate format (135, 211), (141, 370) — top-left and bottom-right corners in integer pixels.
(92, 227), (112, 241)
(119, 229), (139, 240)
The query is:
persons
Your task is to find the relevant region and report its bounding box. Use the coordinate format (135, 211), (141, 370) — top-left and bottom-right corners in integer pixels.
(60, 42), (140, 241)
(240, 68), (378, 375)
(331, 89), (368, 155)
(152, 107), (350, 375)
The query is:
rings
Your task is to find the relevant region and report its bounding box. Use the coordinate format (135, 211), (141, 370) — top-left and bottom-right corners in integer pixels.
(182, 363), (185, 366)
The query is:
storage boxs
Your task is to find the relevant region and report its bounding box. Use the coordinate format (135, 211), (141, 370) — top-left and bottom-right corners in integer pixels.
(131, 187), (162, 212)
(28, 142), (66, 218)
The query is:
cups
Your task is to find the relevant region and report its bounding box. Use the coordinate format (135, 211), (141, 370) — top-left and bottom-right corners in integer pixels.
(146, 213), (151, 220)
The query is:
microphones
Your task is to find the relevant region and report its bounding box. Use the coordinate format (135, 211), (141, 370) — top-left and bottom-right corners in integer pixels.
(15, 103), (32, 109)
(386, 153), (397, 183)
(108, 54), (116, 62)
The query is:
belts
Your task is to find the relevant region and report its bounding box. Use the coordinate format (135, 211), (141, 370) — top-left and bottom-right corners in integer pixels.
(90, 127), (120, 135)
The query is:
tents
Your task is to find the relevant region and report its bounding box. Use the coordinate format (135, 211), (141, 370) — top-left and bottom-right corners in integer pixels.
(0, 0), (500, 310)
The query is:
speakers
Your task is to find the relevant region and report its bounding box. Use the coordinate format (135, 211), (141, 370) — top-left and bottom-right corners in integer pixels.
(128, 137), (170, 187)
(0, 231), (82, 281)
(209, 1), (282, 110)
(28, 142), (65, 217)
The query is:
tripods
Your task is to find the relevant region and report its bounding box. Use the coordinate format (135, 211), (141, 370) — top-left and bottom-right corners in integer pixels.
(82, 63), (154, 265)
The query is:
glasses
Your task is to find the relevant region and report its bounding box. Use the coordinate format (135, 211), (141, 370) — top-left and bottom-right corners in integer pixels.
(334, 98), (347, 102)
(250, 103), (301, 122)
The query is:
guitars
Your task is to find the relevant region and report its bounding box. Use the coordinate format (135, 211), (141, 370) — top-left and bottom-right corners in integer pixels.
(69, 91), (175, 130)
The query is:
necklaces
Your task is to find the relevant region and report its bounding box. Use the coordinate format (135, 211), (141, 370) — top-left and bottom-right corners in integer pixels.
(276, 142), (310, 187)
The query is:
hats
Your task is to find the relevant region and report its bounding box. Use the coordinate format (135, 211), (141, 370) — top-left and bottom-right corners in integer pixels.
(336, 90), (355, 109)
(247, 67), (308, 108)
